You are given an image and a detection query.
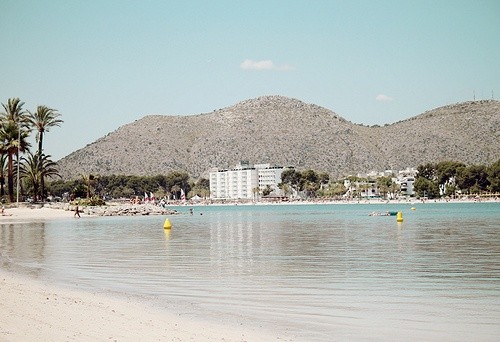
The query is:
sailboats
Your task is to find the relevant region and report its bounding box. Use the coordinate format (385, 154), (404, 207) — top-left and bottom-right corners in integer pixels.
(144, 188), (187, 207)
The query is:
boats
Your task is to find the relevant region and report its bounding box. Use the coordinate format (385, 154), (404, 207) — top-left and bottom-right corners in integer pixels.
(369, 211), (398, 216)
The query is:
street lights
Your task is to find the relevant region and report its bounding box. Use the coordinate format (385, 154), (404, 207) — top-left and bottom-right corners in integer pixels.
(16, 115), (28, 204)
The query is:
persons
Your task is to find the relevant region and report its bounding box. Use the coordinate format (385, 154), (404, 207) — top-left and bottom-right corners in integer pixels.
(1, 205), (6, 216)
(103, 192), (178, 208)
(73, 203), (81, 218)
(373, 194), (499, 204)
